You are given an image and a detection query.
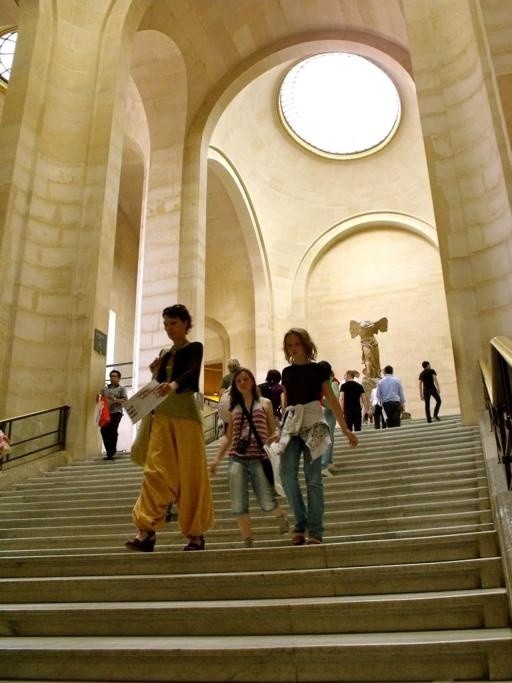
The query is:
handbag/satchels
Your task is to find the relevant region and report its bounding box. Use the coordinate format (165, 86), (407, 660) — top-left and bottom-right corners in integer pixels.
(94, 394), (111, 428)
(263, 442), (286, 498)
(131, 412), (150, 465)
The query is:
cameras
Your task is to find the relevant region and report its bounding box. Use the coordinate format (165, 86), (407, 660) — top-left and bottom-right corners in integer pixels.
(236, 438), (248, 453)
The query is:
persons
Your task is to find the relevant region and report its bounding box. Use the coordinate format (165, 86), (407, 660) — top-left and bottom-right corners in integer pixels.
(97, 304), (214, 552)
(210, 328), (405, 548)
(417, 360), (443, 423)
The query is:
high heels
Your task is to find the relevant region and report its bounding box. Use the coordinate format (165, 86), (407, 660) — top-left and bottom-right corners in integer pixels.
(184, 535), (204, 550)
(126, 530), (156, 552)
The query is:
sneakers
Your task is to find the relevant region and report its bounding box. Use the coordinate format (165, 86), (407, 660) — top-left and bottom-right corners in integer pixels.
(244, 539), (253, 548)
(321, 463), (336, 477)
(279, 513), (320, 545)
